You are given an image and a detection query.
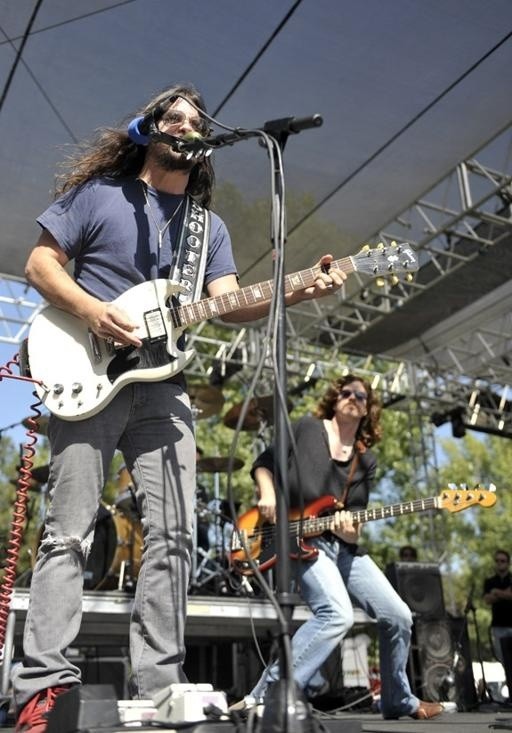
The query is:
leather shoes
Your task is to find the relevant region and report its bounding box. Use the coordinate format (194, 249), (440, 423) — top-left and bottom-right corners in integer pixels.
(384, 699), (445, 719)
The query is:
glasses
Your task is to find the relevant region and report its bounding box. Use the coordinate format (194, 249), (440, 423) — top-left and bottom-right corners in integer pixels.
(163, 110), (209, 136)
(340, 390), (366, 399)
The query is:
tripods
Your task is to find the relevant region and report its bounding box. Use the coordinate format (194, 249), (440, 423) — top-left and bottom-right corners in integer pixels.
(451, 583), (512, 713)
(187, 469), (256, 598)
(107, 507), (142, 593)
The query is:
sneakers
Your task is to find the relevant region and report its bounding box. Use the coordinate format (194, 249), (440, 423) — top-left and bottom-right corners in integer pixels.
(14, 684), (71, 733)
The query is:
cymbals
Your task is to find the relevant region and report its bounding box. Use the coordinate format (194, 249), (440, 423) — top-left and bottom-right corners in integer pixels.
(23, 415), (50, 436)
(30, 465), (49, 483)
(196, 457), (244, 473)
(186, 383), (224, 419)
(224, 395), (294, 431)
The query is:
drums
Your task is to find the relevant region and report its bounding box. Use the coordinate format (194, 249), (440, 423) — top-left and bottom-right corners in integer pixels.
(30, 498), (145, 591)
(117, 462), (137, 508)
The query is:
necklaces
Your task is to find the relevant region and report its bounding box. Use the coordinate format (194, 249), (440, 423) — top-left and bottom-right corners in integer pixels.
(138, 175), (184, 248)
(331, 422), (351, 452)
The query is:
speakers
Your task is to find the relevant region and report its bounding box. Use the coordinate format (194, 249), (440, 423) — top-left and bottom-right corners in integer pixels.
(406, 616), (479, 713)
(387, 561), (445, 618)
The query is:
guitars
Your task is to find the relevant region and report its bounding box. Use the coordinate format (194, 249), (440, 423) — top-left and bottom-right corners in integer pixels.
(232, 480), (496, 576)
(28, 240), (420, 423)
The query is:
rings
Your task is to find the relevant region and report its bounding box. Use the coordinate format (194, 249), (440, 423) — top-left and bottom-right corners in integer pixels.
(325, 283), (334, 289)
(107, 336), (113, 344)
(335, 526), (340, 529)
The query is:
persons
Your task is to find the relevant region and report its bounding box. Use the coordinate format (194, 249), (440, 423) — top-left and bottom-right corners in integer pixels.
(399, 545), (417, 560)
(193, 446), (209, 502)
(480, 550), (511, 667)
(6, 80), (350, 732)
(247, 373), (446, 724)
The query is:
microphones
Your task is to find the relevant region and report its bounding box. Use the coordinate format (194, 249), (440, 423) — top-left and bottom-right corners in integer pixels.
(181, 131), (204, 144)
(431, 405), (465, 425)
(128, 95), (178, 144)
